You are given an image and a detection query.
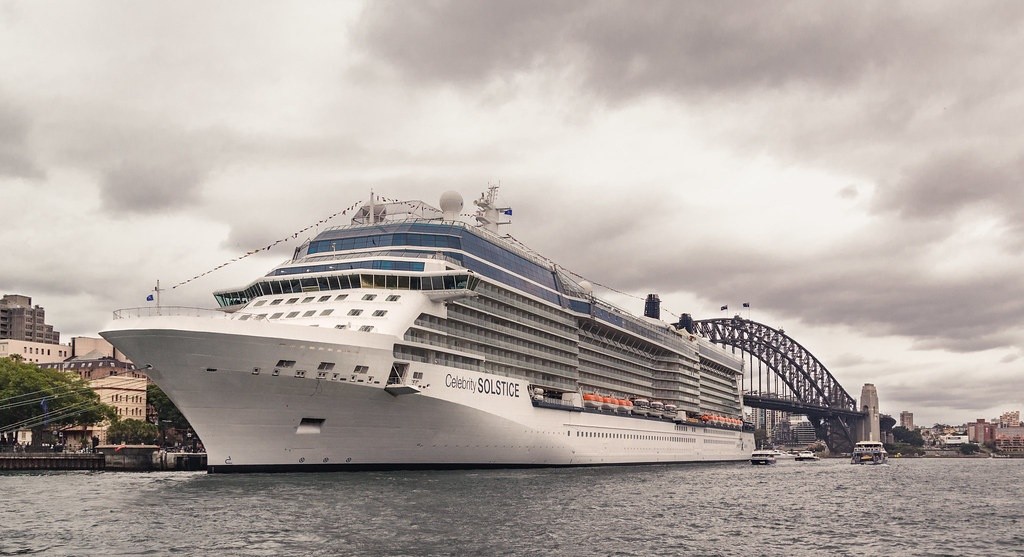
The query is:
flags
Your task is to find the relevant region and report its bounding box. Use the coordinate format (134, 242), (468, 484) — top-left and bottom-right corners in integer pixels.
(743, 302), (749, 307)
(146, 295), (155, 301)
(504, 209), (512, 215)
(721, 305), (728, 311)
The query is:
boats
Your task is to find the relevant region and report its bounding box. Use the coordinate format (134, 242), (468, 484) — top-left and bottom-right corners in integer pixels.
(752, 449), (775, 465)
(774, 450), (784, 456)
(797, 452), (819, 463)
(97, 178), (754, 469)
(851, 441), (888, 465)
